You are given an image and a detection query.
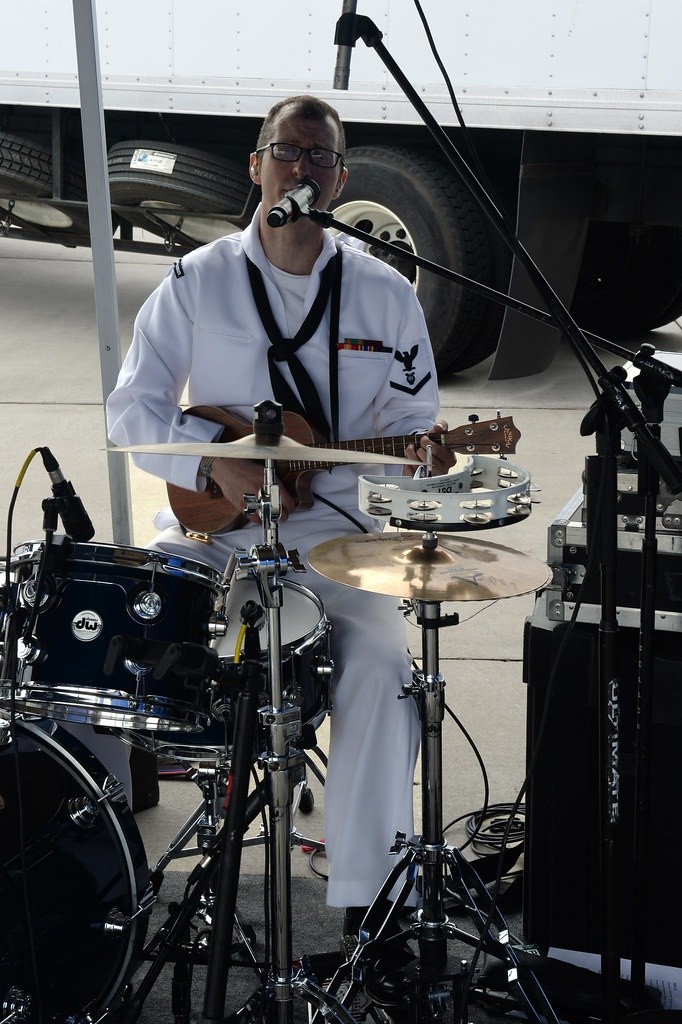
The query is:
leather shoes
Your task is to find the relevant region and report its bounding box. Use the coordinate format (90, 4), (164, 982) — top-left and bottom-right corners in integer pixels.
(341, 908), (420, 1009)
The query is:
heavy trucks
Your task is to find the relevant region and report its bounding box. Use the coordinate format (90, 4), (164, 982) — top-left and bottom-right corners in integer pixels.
(0, 0), (681, 384)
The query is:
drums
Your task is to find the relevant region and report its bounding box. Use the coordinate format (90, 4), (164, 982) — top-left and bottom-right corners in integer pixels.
(107, 570), (338, 761)
(0, 710), (156, 1024)
(0, 539), (231, 733)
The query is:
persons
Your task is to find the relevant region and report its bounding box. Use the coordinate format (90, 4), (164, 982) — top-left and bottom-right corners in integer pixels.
(101, 94), (458, 1006)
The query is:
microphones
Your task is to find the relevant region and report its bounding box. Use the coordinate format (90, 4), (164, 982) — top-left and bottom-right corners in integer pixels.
(267, 179), (323, 228)
(333, 0), (357, 90)
(39, 446), (95, 541)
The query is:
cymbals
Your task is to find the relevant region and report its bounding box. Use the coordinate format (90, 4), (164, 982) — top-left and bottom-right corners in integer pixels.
(102, 433), (434, 469)
(306, 532), (553, 602)
(358, 456), (543, 532)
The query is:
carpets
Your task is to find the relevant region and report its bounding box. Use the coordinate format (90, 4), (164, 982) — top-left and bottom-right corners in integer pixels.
(125, 871), (532, 1023)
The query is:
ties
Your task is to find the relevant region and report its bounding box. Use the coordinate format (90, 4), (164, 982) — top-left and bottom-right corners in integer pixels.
(246, 251), (331, 443)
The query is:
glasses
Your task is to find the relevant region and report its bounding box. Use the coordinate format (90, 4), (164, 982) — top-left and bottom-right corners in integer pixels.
(256, 142), (345, 168)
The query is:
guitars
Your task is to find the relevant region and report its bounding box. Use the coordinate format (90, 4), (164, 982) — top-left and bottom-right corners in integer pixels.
(166, 403), (522, 537)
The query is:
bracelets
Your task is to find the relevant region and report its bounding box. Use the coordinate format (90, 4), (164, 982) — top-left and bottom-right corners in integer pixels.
(200, 456), (217, 478)
